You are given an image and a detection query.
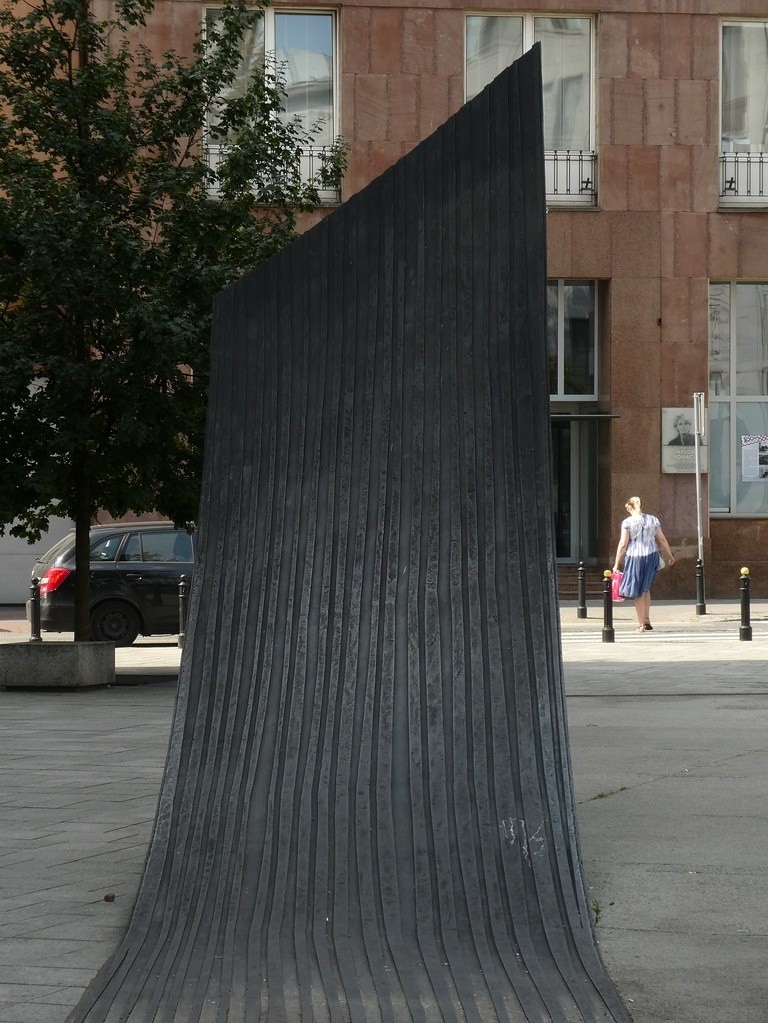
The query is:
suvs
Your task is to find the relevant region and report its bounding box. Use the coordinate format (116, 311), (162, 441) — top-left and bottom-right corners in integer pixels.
(26, 521), (199, 647)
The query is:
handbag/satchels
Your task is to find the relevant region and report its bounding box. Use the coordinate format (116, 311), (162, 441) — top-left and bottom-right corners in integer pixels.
(657, 554), (665, 571)
(611, 570), (625, 602)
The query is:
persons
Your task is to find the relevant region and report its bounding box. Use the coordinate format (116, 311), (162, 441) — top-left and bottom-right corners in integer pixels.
(668, 412), (704, 446)
(612, 496), (676, 633)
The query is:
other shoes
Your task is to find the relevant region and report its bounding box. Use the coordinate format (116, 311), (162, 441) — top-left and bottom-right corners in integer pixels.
(635, 625), (645, 633)
(645, 618), (653, 630)
